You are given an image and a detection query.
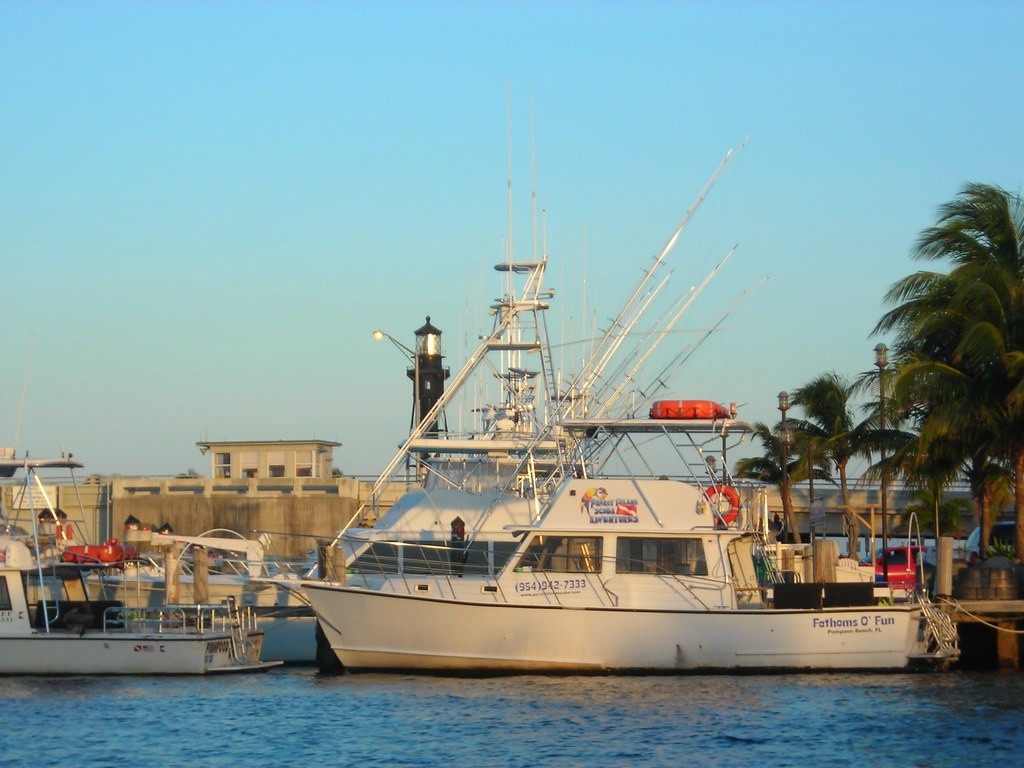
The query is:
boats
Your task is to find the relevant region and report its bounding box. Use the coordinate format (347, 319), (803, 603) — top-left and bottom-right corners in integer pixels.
(299, 261), (960, 673)
(0, 450), (316, 674)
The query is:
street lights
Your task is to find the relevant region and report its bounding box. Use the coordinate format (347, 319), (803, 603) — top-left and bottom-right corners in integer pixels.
(874, 343), (890, 550)
(777, 391), (790, 544)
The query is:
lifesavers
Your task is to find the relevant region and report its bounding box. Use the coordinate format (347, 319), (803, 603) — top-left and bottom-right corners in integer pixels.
(704, 485), (739, 525)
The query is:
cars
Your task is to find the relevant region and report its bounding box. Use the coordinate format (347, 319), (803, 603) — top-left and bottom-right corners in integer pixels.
(861, 544), (927, 591)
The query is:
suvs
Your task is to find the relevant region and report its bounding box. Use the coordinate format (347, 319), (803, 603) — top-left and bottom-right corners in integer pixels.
(963, 521), (1017, 567)
